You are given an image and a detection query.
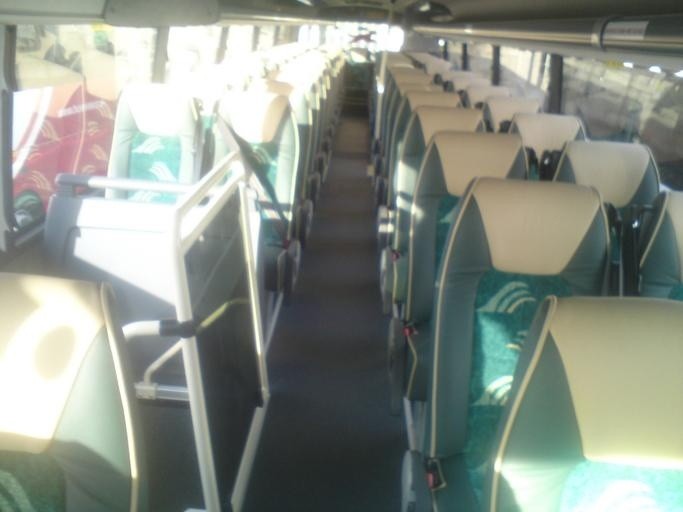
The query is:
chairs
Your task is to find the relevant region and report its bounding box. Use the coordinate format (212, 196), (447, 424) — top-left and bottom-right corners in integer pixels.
(0, 38), (683, 512)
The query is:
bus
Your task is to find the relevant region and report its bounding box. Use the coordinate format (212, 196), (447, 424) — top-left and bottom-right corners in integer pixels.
(13, 23), (123, 228)
